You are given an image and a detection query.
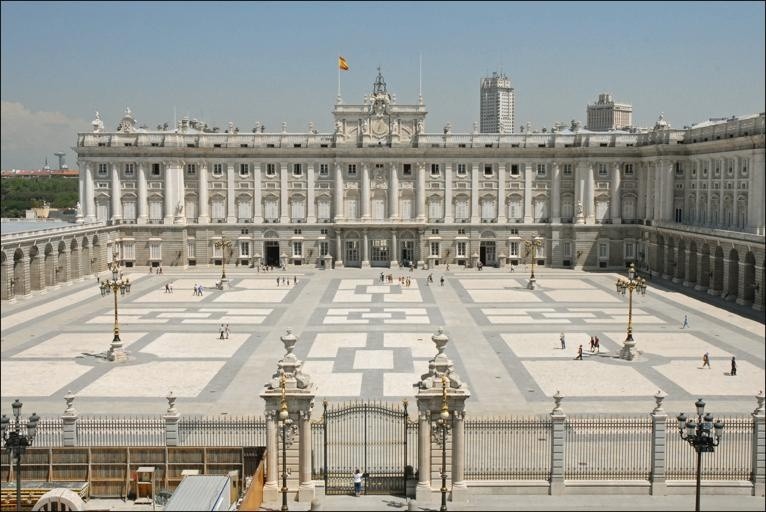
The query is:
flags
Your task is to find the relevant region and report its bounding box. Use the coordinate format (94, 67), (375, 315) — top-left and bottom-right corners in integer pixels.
(339, 56), (350, 72)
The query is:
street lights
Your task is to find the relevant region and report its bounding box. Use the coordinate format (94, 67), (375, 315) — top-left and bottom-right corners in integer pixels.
(100, 267), (131, 341)
(617, 263), (646, 341)
(215, 234), (232, 278)
(0, 400), (39, 510)
(278, 374), (290, 511)
(438, 377), (451, 511)
(524, 234), (541, 277)
(678, 399), (724, 511)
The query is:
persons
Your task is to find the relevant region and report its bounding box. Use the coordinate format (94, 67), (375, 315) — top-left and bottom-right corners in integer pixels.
(576, 345), (584, 360)
(198, 285), (204, 296)
(560, 331), (566, 349)
(589, 336), (595, 352)
(730, 356), (737, 376)
(219, 323), (224, 339)
(680, 314), (690, 329)
(193, 284), (198, 296)
(169, 282), (173, 292)
(149, 265), (163, 275)
(263, 264), (275, 272)
(378, 258), (450, 287)
(165, 282), (170, 293)
(225, 323), (232, 339)
(477, 261), (483, 270)
(702, 352), (712, 369)
(352, 469), (364, 497)
(511, 263), (514, 272)
(276, 275), (297, 286)
(593, 336), (600, 353)
